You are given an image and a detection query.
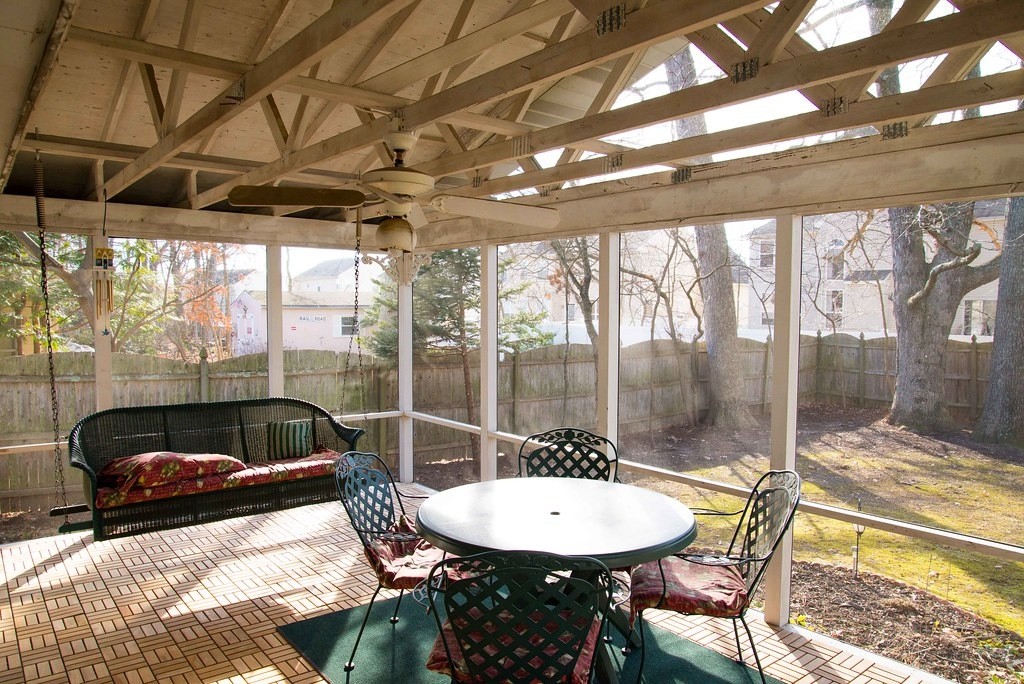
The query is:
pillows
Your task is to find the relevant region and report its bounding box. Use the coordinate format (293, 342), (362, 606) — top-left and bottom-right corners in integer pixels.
(101, 451), (248, 493)
(266, 419), (312, 461)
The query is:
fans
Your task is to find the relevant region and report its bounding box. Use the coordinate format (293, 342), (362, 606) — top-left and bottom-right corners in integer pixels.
(229, 129), (561, 229)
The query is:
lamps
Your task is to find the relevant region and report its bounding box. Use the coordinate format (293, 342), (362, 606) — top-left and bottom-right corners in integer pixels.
(375, 218), (417, 258)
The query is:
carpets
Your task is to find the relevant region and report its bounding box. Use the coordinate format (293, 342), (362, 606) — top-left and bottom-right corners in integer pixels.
(276, 574), (787, 684)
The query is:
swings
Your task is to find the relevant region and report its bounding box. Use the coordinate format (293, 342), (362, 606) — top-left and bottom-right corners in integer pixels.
(28, 147), (381, 544)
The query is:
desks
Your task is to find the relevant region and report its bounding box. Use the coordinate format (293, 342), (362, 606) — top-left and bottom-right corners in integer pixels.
(414, 476), (697, 684)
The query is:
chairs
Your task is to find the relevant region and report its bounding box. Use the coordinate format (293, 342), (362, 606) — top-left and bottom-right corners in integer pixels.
(333, 451), (495, 670)
(621, 470), (801, 684)
(514, 427), (631, 643)
(426, 550), (614, 684)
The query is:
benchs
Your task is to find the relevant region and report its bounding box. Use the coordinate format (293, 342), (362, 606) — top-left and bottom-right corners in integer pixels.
(68, 398), (366, 541)
